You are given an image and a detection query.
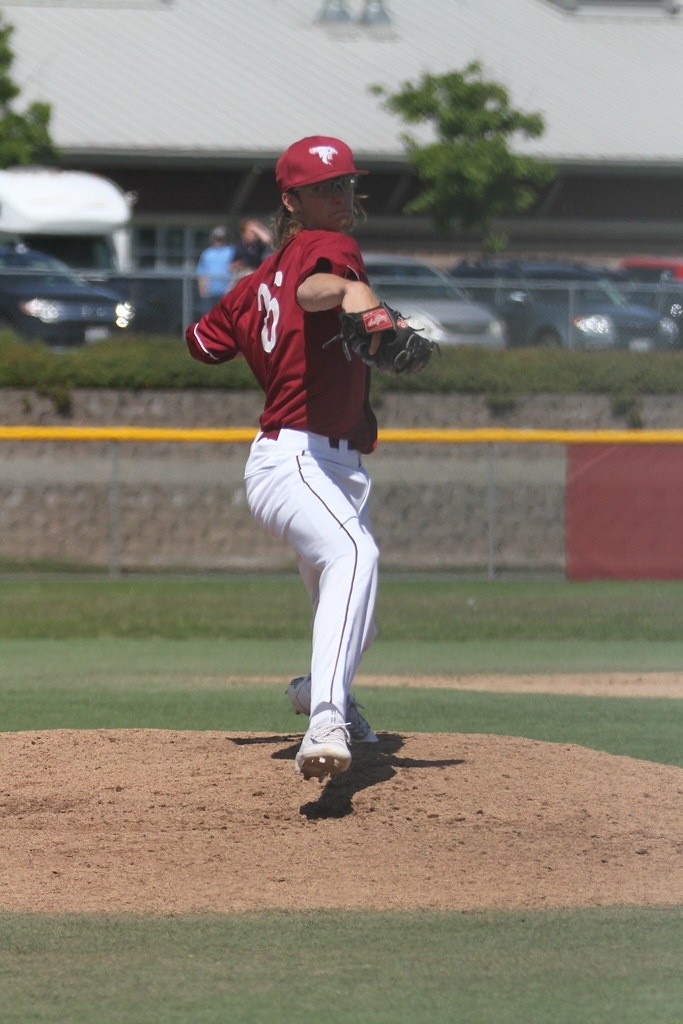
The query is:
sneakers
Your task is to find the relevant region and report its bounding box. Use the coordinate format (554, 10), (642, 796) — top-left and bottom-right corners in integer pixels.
(287, 676), (379, 742)
(295, 720), (351, 783)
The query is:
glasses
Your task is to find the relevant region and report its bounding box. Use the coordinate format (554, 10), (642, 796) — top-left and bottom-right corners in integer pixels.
(288, 176), (352, 196)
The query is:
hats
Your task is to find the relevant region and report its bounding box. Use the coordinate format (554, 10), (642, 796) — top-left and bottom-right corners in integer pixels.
(275, 136), (369, 194)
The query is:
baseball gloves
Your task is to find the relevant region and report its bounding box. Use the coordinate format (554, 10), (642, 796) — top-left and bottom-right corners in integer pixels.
(321, 300), (442, 377)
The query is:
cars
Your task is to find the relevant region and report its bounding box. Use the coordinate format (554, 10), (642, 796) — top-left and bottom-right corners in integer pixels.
(0, 245), (136, 346)
(448, 261), (683, 350)
(360, 252), (507, 348)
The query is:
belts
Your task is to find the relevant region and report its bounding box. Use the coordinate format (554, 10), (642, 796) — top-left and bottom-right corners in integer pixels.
(256, 429), (354, 449)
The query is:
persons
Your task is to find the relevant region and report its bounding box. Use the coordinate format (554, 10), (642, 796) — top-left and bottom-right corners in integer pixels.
(184, 136), (443, 784)
(194, 226), (237, 298)
(231, 219), (275, 273)
